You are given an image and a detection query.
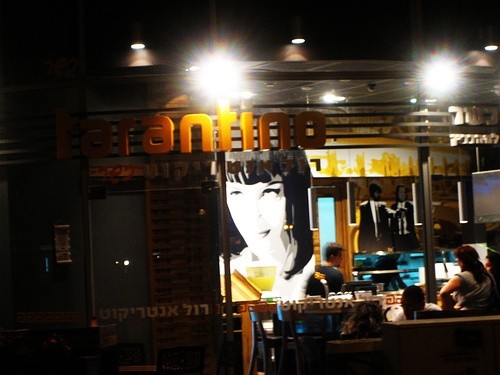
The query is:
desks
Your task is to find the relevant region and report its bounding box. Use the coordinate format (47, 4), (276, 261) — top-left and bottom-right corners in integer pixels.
(381, 315), (500, 375)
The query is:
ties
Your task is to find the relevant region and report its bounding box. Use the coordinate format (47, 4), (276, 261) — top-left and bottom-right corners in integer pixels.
(375, 206), (381, 237)
(401, 203), (404, 236)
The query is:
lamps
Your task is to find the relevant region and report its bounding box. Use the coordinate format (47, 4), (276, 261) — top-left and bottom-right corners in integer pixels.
(484, 31), (499, 51)
(291, 17), (305, 44)
(131, 31), (146, 50)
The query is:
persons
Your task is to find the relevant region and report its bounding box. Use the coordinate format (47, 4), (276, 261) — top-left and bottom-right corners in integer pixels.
(341, 302), (382, 338)
(318, 243), (344, 293)
(440, 245), (496, 310)
(389, 185), (420, 251)
(359, 183), (400, 253)
(484, 252), (500, 309)
(392, 285), (442, 321)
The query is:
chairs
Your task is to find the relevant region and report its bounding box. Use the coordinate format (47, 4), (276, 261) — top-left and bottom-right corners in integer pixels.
(248, 284), (481, 375)
